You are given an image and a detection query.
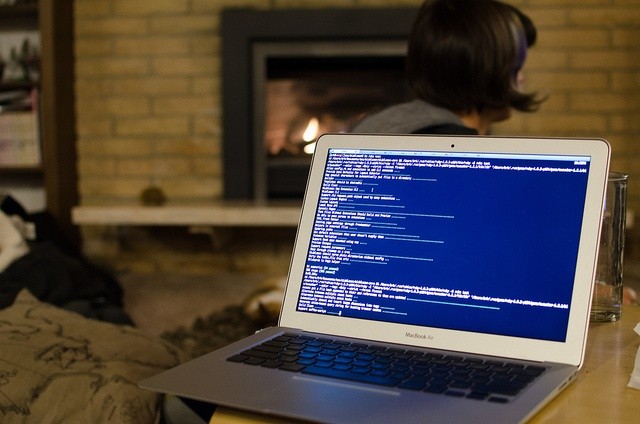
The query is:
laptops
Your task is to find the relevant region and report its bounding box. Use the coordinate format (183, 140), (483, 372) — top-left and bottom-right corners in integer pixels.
(134, 132), (612, 424)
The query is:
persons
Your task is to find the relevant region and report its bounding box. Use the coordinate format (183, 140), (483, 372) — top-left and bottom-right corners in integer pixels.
(348, 0), (639, 311)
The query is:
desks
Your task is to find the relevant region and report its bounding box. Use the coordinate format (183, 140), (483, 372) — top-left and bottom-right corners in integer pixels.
(207, 278), (638, 424)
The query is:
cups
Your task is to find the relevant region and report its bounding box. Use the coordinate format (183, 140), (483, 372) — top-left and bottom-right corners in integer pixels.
(589, 171), (629, 323)
